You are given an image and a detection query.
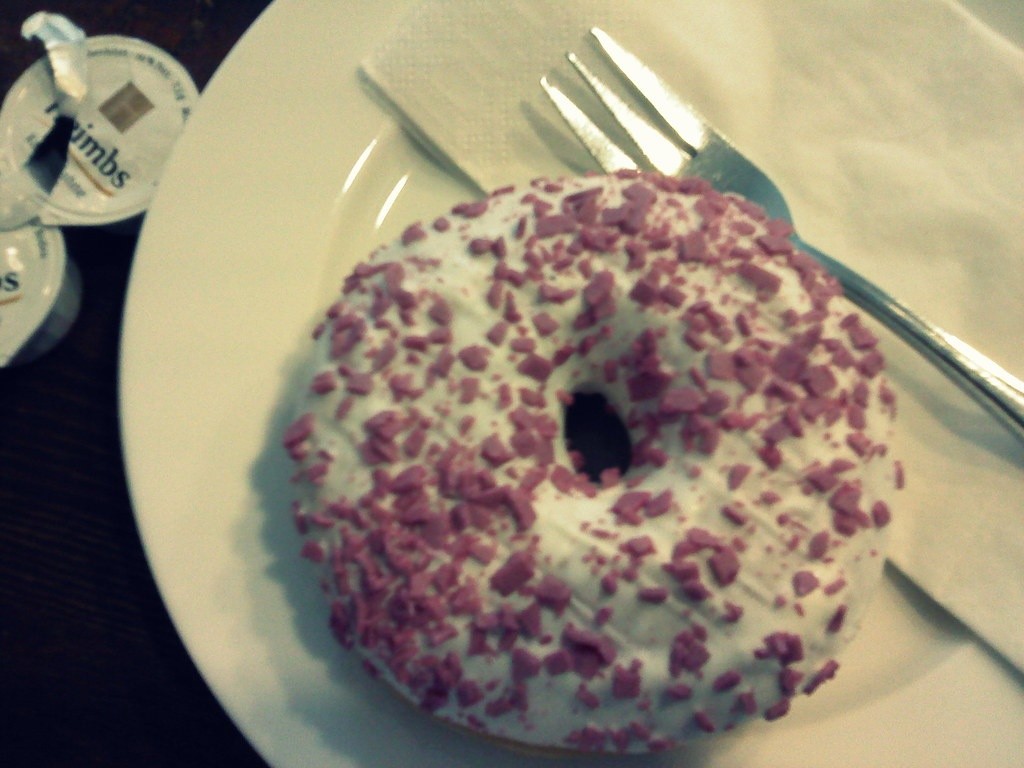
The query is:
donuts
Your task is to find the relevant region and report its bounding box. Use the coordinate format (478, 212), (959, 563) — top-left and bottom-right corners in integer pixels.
(281, 168), (906, 758)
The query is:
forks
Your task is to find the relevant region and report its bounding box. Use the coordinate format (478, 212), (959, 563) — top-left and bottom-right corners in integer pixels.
(544, 24), (1024, 446)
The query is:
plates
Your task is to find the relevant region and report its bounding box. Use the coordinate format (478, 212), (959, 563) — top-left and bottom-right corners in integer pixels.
(114, 1), (1023, 768)
